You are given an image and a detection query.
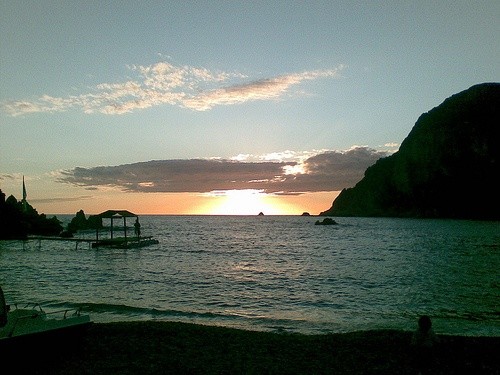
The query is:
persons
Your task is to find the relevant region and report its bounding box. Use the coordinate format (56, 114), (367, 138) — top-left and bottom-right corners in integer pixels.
(134, 220), (141, 238)
(411, 315), (440, 374)
